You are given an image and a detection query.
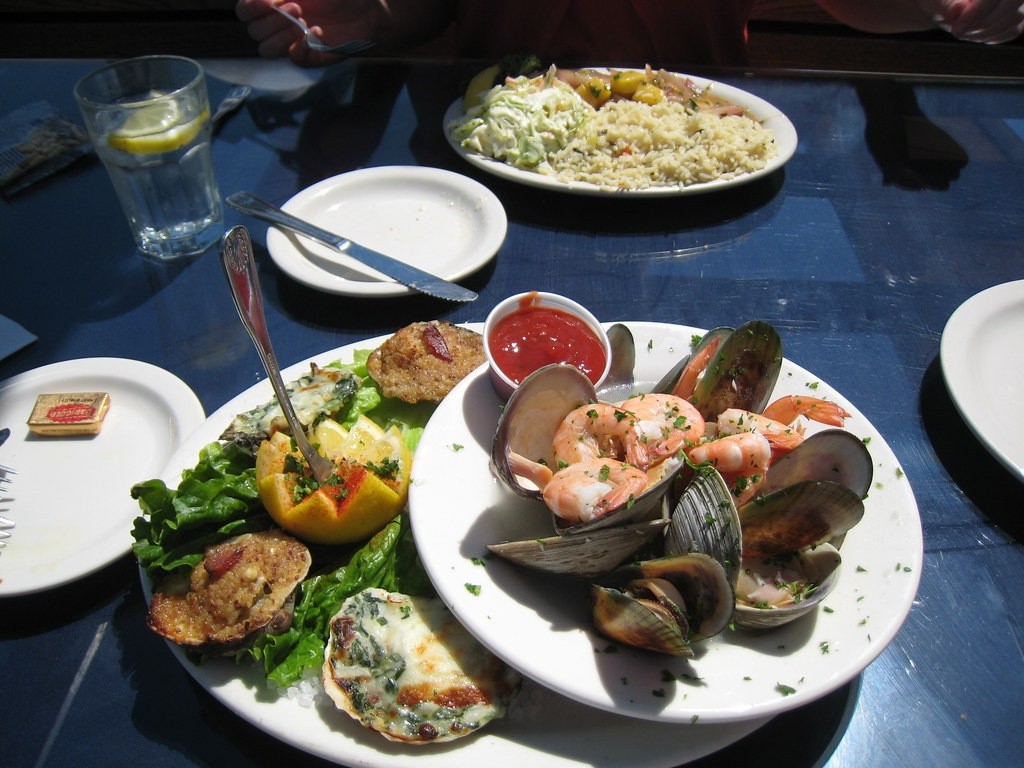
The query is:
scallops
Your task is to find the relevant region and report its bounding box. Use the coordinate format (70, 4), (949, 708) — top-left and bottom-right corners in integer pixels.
(322, 587), (512, 747)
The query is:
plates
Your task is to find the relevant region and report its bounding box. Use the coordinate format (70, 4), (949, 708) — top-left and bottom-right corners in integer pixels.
(135, 334), (781, 768)
(407, 320), (923, 724)
(266, 164), (508, 299)
(443, 66), (798, 198)
(940, 279), (1024, 487)
(0, 356), (205, 595)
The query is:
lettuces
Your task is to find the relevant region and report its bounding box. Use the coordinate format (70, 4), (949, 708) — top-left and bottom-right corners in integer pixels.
(129, 348), (427, 690)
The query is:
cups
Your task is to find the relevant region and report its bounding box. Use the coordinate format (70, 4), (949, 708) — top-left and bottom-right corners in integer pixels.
(73, 54), (225, 264)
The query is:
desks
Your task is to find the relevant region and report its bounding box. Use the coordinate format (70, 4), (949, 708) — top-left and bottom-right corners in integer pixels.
(0, 52), (1024, 768)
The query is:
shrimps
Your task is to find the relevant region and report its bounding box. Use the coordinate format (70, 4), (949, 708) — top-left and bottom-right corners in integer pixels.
(542, 335), (853, 526)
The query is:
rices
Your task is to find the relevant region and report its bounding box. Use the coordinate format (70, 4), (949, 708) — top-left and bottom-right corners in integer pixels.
(561, 102), (777, 189)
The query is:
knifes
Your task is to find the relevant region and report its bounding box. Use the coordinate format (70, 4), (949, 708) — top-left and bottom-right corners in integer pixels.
(224, 189), (480, 303)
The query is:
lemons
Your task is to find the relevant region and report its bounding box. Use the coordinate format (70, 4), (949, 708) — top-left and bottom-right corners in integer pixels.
(109, 92), (210, 156)
(257, 416), (412, 542)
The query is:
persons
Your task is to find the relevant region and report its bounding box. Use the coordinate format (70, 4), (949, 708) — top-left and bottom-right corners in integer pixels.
(234, 0), (1024, 79)
